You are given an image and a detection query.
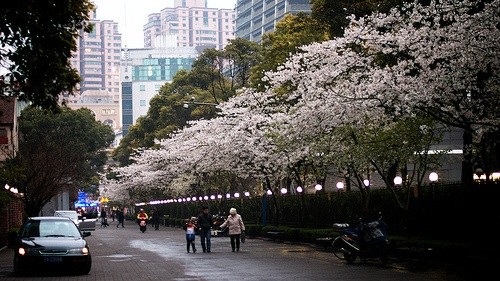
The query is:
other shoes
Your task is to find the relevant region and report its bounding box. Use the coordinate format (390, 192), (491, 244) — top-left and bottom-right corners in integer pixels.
(187, 252), (189, 253)
(193, 248), (197, 251)
(122, 226), (124, 227)
(232, 251), (235, 252)
(116, 226), (118, 227)
(207, 250), (210, 252)
(203, 250), (205, 252)
(237, 248), (239, 251)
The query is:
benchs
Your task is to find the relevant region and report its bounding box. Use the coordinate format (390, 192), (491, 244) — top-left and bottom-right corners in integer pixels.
(316, 238), (333, 250)
(266, 231), (285, 244)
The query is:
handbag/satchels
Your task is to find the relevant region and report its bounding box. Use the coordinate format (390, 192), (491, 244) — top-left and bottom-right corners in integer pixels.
(241, 235), (245, 243)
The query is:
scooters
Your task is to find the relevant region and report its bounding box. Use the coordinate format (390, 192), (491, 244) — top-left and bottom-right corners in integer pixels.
(332, 211), (391, 265)
(139, 217), (148, 233)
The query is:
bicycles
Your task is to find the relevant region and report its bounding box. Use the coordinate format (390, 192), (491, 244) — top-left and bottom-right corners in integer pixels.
(332, 236), (346, 260)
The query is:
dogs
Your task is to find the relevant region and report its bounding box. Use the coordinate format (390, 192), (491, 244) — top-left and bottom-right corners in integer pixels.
(100, 222), (109, 228)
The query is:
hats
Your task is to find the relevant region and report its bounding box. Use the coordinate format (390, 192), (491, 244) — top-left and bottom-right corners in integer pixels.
(140, 209), (144, 211)
(230, 208), (236, 214)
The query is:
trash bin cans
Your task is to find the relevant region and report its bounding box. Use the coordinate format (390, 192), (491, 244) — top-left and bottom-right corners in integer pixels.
(164, 215), (169, 227)
(8, 228), (20, 249)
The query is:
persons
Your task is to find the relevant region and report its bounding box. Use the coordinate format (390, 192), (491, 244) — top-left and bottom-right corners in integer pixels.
(183, 219), (199, 253)
(76, 206), (125, 228)
(217, 208), (246, 253)
(150, 209), (160, 231)
(196, 206), (217, 253)
(136, 208), (148, 226)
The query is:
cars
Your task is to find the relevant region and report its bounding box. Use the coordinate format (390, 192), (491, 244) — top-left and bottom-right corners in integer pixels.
(12, 216), (92, 275)
(53, 210), (96, 233)
